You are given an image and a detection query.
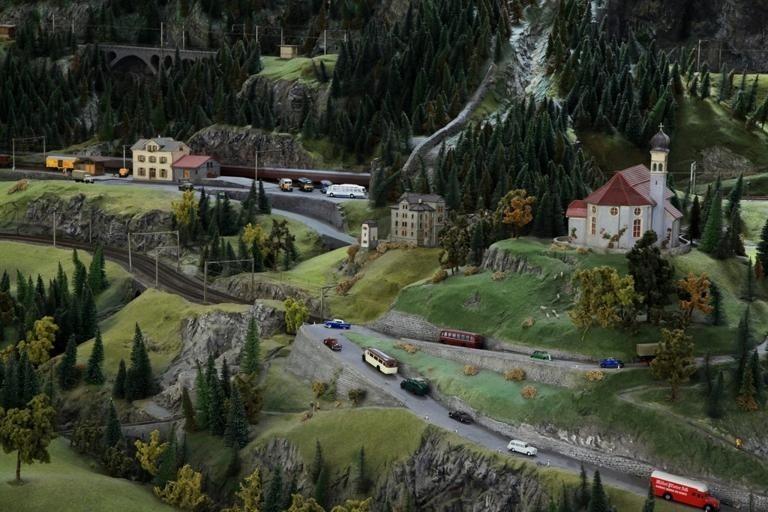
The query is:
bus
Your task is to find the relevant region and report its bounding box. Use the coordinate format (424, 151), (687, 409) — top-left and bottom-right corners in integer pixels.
(439, 329), (484, 348)
(362, 347), (399, 375)
(326, 184), (367, 198)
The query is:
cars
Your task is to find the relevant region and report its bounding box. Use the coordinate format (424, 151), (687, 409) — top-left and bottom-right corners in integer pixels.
(530, 351), (549, 360)
(599, 357), (624, 368)
(449, 411), (474, 425)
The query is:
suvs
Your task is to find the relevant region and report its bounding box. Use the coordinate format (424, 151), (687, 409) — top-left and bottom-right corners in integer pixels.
(400, 378), (429, 396)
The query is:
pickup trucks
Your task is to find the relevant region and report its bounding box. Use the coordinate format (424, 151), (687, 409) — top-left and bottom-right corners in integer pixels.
(324, 338), (342, 351)
(324, 319), (351, 329)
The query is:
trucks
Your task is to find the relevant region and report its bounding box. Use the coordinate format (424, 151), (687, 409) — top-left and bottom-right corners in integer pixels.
(297, 178), (314, 192)
(320, 180), (333, 192)
(72, 169), (94, 184)
(635, 343), (666, 367)
(279, 178), (293, 192)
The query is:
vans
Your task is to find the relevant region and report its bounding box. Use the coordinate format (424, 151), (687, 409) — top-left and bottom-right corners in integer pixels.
(650, 470), (721, 512)
(507, 440), (538, 456)
